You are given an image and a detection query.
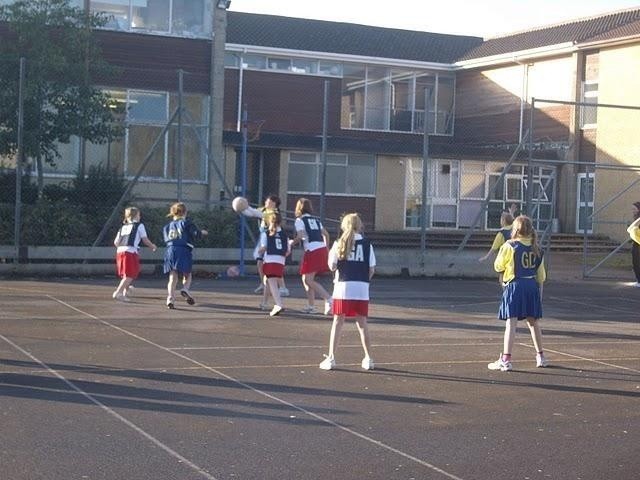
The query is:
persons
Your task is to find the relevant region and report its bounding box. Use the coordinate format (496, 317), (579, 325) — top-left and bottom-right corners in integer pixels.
(487, 215), (547, 371)
(241, 195), (290, 297)
(256, 211), (292, 316)
(627, 217), (640, 244)
(162, 202), (208, 309)
(478, 202), (518, 285)
(112, 206), (157, 300)
(289, 197), (333, 316)
(629, 201), (640, 288)
(319, 212), (377, 371)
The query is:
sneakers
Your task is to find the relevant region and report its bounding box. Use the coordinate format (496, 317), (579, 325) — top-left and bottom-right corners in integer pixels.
(535, 356), (548, 368)
(362, 358), (374, 370)
(112, 283), (333, 317)
(487, 352), (512, 372)
(319, 354), (336, 370)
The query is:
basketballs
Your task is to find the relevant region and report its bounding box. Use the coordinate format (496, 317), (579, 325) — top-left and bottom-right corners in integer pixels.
(227, 266), (240, 279)
(231, 197), (248, 212)
